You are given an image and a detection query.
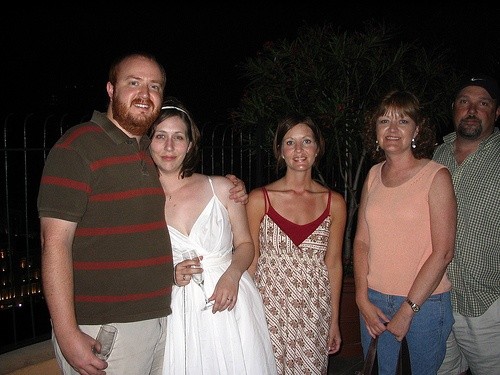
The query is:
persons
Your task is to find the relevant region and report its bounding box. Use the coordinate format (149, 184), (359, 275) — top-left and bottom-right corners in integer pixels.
(245, 116), (347, 375)
(431, 79), (500, 375)
(37, 53), (249, 375)
(353, 88), (457, 375)
(147, 98), (278, 375)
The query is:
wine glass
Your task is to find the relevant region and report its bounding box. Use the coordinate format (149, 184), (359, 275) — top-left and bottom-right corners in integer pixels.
(183, 250), (214, 311)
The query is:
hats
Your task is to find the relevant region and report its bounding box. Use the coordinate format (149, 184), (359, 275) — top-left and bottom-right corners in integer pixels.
(454, 71), (499, 105)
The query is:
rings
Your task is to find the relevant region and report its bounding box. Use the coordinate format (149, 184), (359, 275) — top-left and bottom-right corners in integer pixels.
(227, 298), (233, 302)
(183, 275), (186, 281)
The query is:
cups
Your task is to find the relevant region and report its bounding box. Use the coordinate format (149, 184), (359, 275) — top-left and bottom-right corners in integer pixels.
(93, 324), (118, 362)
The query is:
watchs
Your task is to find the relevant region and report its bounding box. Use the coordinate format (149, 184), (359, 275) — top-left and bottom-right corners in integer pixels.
(405, 297), (421, 314)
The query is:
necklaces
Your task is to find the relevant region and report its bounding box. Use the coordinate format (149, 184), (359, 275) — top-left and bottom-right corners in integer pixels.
(161, 180), (177, 200)
